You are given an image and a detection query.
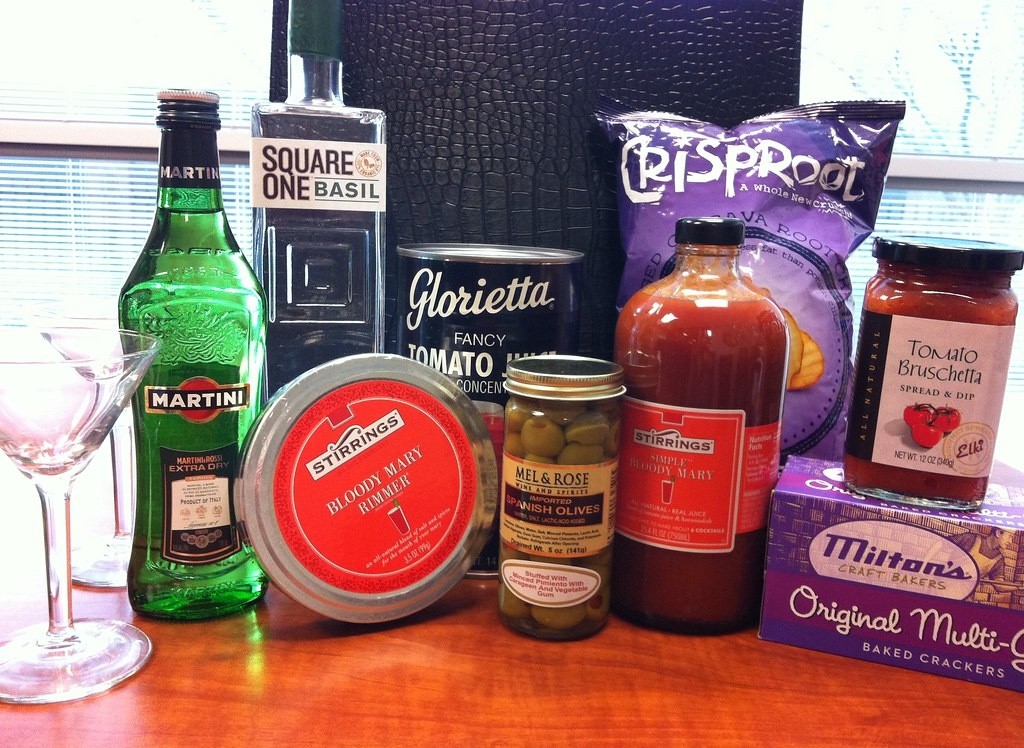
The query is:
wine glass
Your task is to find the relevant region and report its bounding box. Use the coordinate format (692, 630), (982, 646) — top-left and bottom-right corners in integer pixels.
(0, 323), (165, 708)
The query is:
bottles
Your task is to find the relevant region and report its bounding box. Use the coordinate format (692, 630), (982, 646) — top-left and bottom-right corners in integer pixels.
(616, 214), (793, 634)
(249, 50), (386, 404)
(116, 86), (275, 624)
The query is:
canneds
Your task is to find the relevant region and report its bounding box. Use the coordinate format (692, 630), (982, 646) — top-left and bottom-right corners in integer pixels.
(394, 243), (585, 579)
(844, 234), (1024, 510)
(500, 356), (627, 642)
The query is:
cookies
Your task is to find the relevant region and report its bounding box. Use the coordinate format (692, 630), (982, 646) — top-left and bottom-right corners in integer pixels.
(783, 307), (824, 388)
